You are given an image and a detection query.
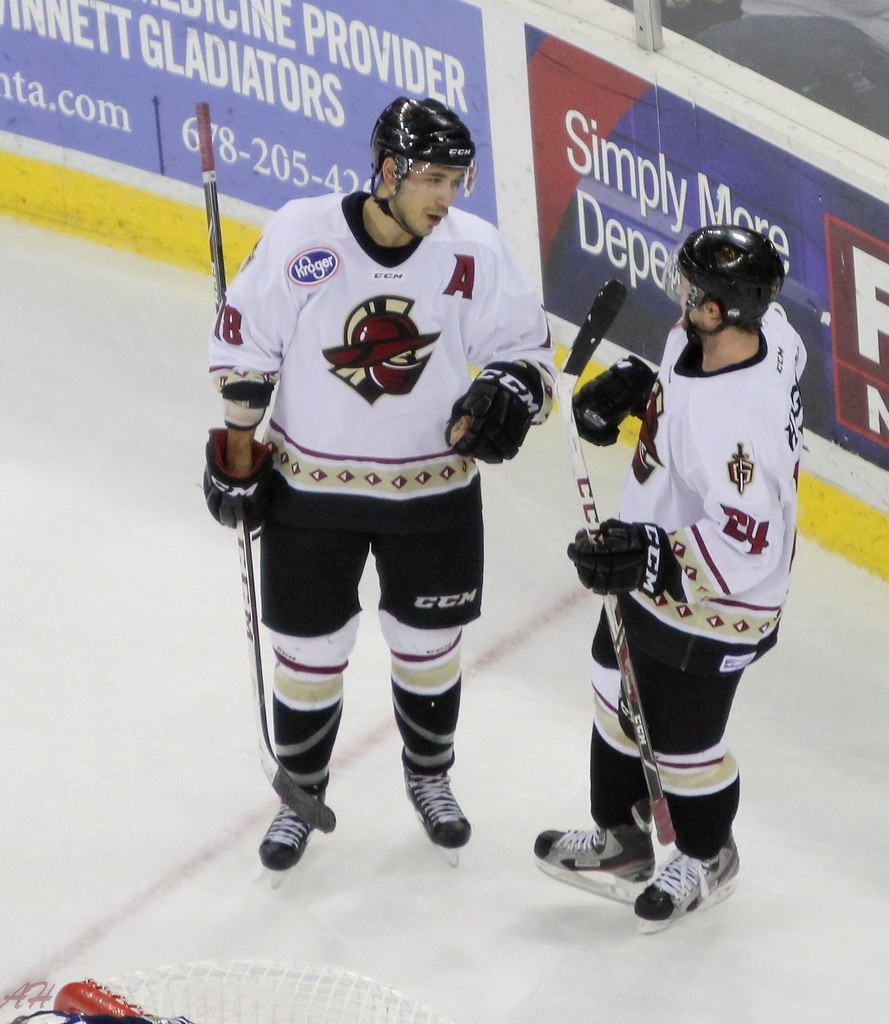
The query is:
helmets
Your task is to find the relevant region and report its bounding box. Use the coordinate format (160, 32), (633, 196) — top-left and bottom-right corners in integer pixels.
(677, 225), (786, 327)
(371, 96), (476, 176)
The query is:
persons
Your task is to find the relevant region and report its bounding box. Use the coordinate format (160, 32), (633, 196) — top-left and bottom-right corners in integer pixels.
(532, 225), (806, 933)
(202, 97), (552, 887)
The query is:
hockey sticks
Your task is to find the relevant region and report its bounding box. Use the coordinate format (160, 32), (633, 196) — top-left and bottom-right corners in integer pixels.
(195, 102), (338, 836)
(553, 278), (678, 845)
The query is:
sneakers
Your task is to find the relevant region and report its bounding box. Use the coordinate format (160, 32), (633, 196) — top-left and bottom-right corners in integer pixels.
(402, 764), (471, 868)
(259, 787), (326, 887)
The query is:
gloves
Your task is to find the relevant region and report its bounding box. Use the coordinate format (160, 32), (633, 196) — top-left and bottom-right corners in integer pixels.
(571, 354), (656, 447)
(566, 519), (669, 597)
(444, 361), (544, 465)
(203, 427), (276, 530)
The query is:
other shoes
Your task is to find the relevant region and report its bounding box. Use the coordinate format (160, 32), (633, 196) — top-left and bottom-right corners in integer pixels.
(533, 821), (657, 903)
(633, 825), (740, 933)
